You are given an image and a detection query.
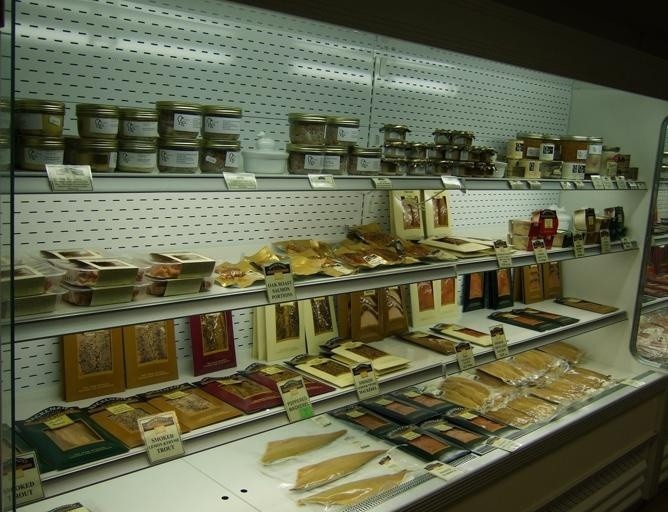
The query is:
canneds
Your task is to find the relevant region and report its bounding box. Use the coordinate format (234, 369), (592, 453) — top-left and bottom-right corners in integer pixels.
(0, 98), (243, 174)
(285, 112), (498, 177)
(660, 152), (668, 181)
(506, 132), (639, 180)
